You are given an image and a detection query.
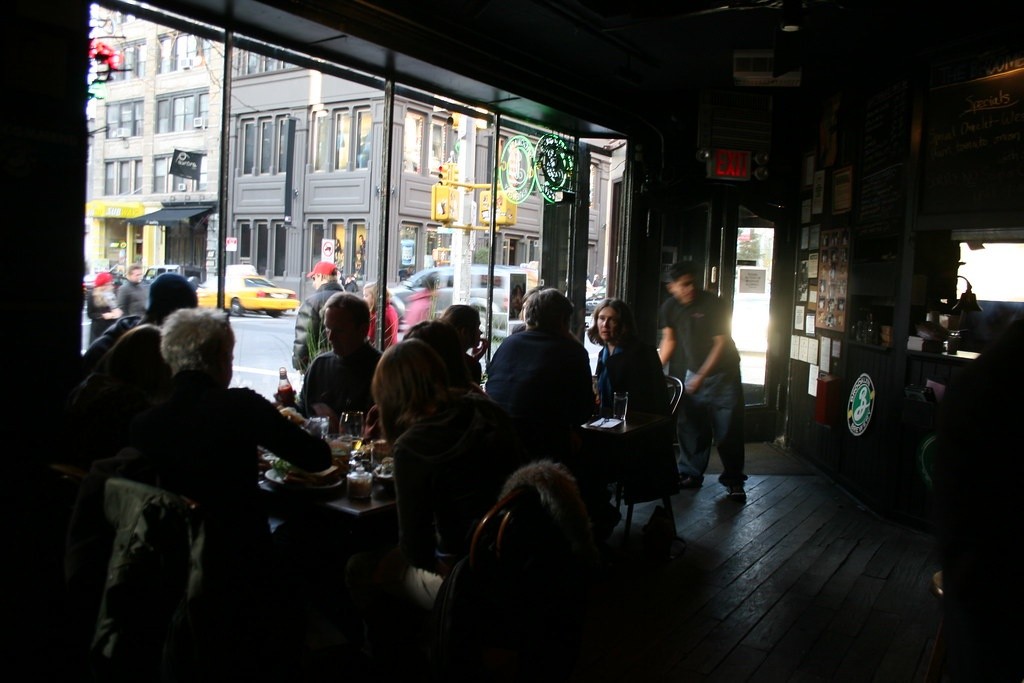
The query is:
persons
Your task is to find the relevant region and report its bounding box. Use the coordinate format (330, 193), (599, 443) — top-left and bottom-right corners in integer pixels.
(656, 260), (748, 501)
(359, 235), (366, 275)
(67, 265), (672, 683)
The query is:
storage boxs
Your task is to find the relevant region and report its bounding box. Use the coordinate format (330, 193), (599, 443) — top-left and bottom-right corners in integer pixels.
(831, 165), (853, 216)
(923, 340), (944, 355)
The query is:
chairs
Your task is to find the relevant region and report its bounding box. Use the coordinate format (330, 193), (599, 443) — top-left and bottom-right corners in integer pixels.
(616, 375), (688, 565)
(87, 476), (214, 683)
(430, 489), (592, 683)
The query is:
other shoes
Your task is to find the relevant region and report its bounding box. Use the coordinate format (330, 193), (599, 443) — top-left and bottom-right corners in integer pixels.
(729, 483), (746, 500)
(679, 475), (704, 489)
(597, 504), (622, 542)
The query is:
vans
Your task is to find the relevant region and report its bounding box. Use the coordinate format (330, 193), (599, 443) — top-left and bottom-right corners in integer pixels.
(354, 265), (538, 340)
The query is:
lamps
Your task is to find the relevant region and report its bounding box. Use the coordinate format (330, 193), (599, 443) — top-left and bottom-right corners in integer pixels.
(947, 291), (984, 355)
(696, 144), (771, 183)
(778, 11), (806, 33)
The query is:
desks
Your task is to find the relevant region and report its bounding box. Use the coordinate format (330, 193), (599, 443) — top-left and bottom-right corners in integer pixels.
(582, 409), (672, 441)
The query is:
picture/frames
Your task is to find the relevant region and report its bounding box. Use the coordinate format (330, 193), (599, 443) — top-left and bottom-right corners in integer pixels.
(810, 168), (827, 215)
(808, 223), (821, 251)
(800, 151), (817, 190)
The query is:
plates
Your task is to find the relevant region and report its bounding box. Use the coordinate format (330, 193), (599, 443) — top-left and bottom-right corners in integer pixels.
(265, 469), (342, 489)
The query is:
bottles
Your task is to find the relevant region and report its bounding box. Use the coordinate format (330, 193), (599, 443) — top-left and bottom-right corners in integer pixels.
(591, 375), (599, 414)
(852, 320), (877, 345)
(278, 367), (294, 407)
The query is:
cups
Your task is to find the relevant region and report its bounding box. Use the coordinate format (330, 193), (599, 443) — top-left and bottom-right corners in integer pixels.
(347, 471), (372, 498)
(310, 416), (329, 439)
(614, 391), (628, 419)
(372, 440), (391, 466)
(947, 335), (961, 354)
(339, 410), (365, 438)
(326, 433), (352, 465)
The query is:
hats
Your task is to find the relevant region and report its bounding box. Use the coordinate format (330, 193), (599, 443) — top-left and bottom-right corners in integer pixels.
(93, 273), (113, 288)
(306, 262), (339, 280)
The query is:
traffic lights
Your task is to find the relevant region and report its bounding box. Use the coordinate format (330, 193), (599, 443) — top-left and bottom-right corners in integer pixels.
(451, 163), (460, 190)
(437, 161), (451, 188)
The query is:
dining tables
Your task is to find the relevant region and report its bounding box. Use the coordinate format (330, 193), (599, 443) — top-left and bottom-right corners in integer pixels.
(312, 484), (397, 520)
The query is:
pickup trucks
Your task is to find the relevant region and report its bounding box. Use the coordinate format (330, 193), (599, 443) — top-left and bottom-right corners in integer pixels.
(140, 264), (183, 287)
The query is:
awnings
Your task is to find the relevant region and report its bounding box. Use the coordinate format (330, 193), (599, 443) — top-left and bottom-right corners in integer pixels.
(123, 206), (212, 229)
(84, 199), (144, 218)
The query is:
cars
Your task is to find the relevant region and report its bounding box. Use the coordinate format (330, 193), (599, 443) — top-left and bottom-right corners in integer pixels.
(82, 273), (98, 298)
(194, 265), (301, 318)
(586, 277), (607, 331)
